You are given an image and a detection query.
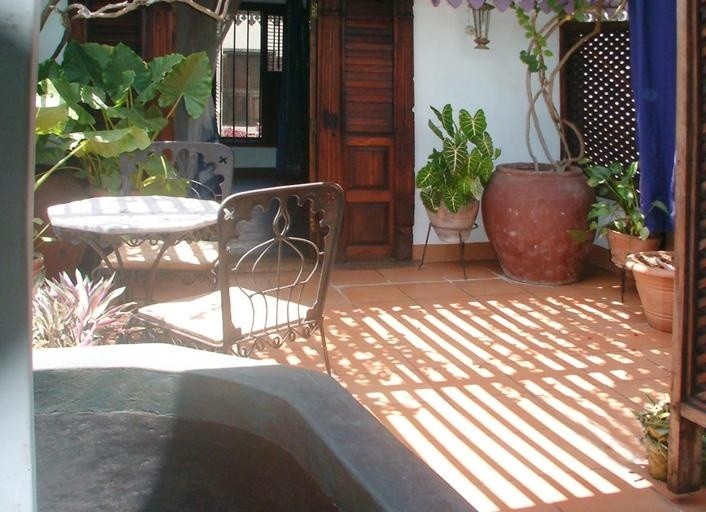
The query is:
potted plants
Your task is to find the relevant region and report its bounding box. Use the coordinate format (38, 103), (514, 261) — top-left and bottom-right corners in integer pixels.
(570, 159), (667, 270)
(417, 104), (502, 242)
(625, 250), (673, 332)
(633, 404), (669, 481)
(482, 0), (626, 283)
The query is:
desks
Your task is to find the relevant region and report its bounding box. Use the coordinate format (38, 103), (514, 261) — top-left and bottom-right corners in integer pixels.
(45, 195), (229, 305)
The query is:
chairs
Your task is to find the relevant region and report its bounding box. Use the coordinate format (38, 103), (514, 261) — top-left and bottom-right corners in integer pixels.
(136, 181), (345, 375)
(101, 138), (236, 306)
